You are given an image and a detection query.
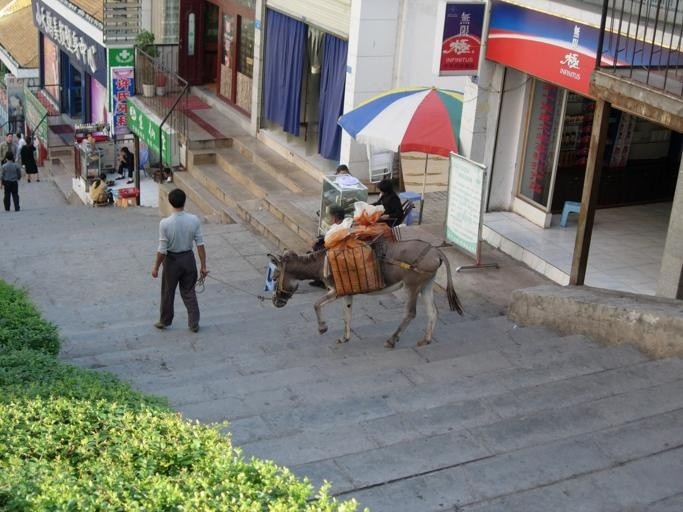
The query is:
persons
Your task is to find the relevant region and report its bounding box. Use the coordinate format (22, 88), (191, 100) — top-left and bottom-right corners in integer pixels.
(368, 181), (404, 227)
(0, 133), (39, 211)
(90, 174), (113, 204)
(336, 165), (352, 176)
(152, 188), (206, 332)
(326, 204), (353, 241)
(115, 147), (133, 184)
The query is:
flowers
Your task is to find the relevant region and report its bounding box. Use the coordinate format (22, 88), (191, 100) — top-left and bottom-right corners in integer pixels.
(155, 74), (166, 86)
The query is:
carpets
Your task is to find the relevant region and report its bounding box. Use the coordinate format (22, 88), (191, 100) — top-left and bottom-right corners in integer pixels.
(161, 93), (212, 111)
(49, 124), (73, 134)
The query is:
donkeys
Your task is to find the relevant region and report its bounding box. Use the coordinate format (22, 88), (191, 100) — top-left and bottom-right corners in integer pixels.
(266, 239), (464, 348)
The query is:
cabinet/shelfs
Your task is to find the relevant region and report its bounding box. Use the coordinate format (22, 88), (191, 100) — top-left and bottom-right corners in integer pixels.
(553, 90), (682, 214)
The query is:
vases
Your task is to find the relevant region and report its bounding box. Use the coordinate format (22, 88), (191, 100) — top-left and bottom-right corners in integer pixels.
(155, 86), (165, 96)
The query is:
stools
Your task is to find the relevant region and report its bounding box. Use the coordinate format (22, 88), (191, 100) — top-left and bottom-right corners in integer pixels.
(560, 200), (581, 228)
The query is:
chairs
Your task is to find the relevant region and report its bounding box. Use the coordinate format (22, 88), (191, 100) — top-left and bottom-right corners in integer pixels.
(138, 151), (149, 177)
(92, 192), (110, 207)
(390, 199), (415, 240)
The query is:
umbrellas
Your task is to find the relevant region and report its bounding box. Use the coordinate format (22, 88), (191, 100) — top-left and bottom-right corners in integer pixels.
(336, 86), (464, 225)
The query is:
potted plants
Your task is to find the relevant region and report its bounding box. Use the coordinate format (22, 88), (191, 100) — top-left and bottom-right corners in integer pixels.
(134, 29), (161, 97)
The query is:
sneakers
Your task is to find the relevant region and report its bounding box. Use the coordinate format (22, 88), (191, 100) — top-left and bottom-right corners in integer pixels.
(127, 179), (134, 184)
(154, 322), (172, 330)
(190, 325), (200, 333)
(115, 175), (125, 180)
